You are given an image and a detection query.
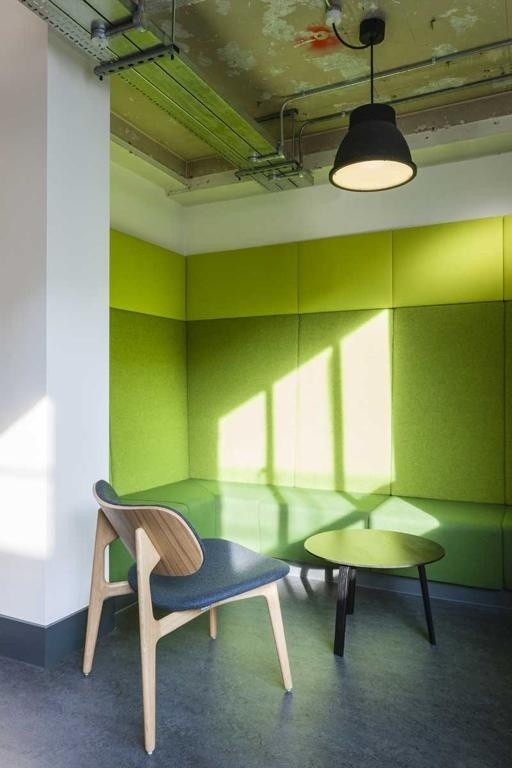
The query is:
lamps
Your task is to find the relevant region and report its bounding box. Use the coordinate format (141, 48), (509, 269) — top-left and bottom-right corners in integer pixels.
(328, 18), (417, 192)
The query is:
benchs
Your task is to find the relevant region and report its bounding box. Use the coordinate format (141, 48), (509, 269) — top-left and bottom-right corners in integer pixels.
(109, 478), (512, 590)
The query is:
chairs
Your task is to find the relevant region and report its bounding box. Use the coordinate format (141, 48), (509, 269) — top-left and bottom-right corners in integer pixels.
(81, 479), (293, 757)
